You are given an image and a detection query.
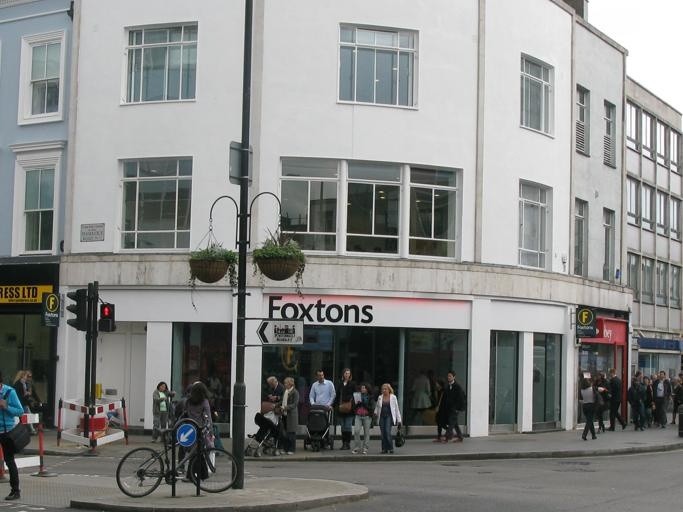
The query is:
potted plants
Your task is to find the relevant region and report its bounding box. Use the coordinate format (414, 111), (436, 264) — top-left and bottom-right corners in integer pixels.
(186, 218), (236, 307)
(250, 218), (309, 299)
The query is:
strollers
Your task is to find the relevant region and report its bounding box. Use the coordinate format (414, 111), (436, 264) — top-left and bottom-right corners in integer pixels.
(300, 403), (336, 453)
(244, 408), (287, 458)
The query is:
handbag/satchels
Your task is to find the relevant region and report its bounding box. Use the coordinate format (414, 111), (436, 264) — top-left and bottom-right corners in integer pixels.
(338, 400), (352, 414)
(593, 400), (609, 412)
(5, 422), (31, 455)
(395, 427), (405, 448)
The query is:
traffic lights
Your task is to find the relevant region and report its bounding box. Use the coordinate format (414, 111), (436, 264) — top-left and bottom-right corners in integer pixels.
(63, 288), (89, 333)
(97, 302), (117, 333)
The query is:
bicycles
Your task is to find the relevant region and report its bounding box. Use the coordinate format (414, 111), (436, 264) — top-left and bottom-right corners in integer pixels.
(111, 410), (242, 499)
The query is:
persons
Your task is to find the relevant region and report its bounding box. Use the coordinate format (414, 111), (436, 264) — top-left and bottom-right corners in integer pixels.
(23, 370), (43, 413)
(12, 368), (38, 435)
(153, 381), (215, 476)
(262, 375), (300, 455)
(575, 366), (682, 441)
(0, 372), (31, 501)
(309, 367), (401, 454)
(411, 368), (467, 444)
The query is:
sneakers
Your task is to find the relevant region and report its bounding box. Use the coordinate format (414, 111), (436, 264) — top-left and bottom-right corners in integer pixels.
(5, 490), (21, 500)
(591, 436), (597, 439)
(174, 470), (195, 483)
(432, 438), (463, 443)
(381, 449), (394, 455)
(596, 419), (676, 434)
(279, 449), (295, 455)
(582, 434), (588, 440)
(351, 448), (369, 455)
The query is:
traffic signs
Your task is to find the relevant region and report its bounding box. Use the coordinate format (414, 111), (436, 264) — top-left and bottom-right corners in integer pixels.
(257, 319), (304, 345)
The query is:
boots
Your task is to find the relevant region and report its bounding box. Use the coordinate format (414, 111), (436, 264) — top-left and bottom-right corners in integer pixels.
(339, 430), (352, 450)
